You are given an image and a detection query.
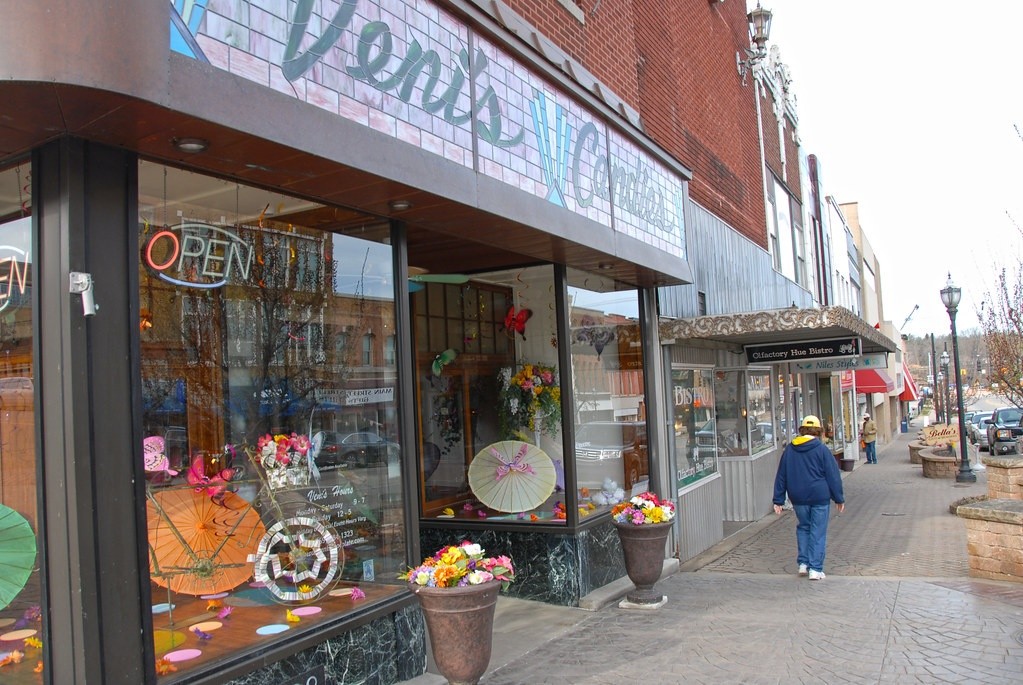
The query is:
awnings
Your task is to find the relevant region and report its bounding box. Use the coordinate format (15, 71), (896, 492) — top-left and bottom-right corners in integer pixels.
(899, 360), (917, 401)
(660, 305), (897, 353)
(855, 369), (894, 393)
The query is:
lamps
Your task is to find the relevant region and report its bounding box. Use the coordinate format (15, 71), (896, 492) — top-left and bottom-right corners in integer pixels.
(736, 0), (773, 83)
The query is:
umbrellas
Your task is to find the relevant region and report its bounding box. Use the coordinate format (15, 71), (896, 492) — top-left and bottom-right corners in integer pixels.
(146, 484), (266, 596)
(1, 504), (35, 609)
(468, 440), (557, 514)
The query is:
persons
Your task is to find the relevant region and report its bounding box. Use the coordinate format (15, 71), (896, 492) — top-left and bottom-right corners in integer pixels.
(772, 416), (845, 580)
(861, 413), (877, 464)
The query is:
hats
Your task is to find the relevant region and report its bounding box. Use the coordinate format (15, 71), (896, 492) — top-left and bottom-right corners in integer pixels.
(863, 413), (870, 418)
(801, 415), (820, 427)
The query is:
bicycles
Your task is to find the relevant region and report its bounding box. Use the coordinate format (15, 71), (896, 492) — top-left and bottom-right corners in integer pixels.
(146, 438), (347, 606)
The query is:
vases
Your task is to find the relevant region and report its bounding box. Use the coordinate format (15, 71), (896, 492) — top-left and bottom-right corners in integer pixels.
(407, 578), (503, 685)
(526, 395), (556, 420)
(840, 459), (855, 472)
(265, 465), (313, 492)
(610, 519), (676, 603)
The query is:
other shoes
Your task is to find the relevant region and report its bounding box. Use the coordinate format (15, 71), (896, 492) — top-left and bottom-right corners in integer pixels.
(864, 462), (869, 464)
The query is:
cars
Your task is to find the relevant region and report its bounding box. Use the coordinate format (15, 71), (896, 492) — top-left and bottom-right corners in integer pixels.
(313, 432), (400, 471)
(767, 387), (801, 411)
(965, 406), (1023, 457)
(149, 426), (188, 457)
(685, 416), (762, 468)
(756, 423), (783, 443)
(575, 420), (649, 490)
(223, 446), (269, 480)
(782, 419), (795, 437)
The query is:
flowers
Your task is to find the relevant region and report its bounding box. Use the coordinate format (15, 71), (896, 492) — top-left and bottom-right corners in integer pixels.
(497, 361), (562, 440)
(0, 577), (370, 677)
(443, 476), (626, 521)
(256, 431), (313, 469)
(610, 490), (676, 525)
(393, 540), (515, 594)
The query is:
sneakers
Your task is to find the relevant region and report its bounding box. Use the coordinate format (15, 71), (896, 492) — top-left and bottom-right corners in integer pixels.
(799, 564), (808, 574)
(809, 569), (826, 579)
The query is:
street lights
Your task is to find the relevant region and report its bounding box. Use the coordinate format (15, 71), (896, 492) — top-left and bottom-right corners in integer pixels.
(939, 341), (951, 427)
(939, 272), (976, 482)
(937, 372), (946, 424)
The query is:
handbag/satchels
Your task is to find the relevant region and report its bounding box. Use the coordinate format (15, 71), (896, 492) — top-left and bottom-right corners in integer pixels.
(861, 440), (866, 448)
